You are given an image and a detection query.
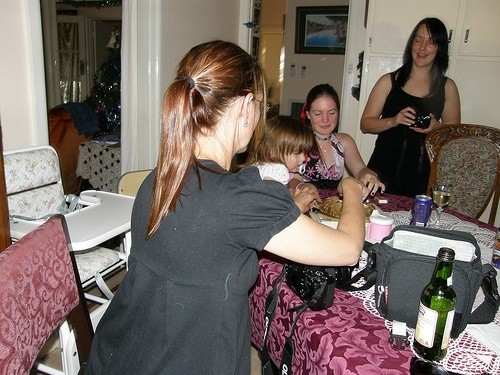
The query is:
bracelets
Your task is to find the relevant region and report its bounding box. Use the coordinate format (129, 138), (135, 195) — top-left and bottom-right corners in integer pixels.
(296, 182), (303, 190)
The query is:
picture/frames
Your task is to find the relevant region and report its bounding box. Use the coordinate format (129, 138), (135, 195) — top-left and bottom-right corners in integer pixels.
(295, 6), (349, 55)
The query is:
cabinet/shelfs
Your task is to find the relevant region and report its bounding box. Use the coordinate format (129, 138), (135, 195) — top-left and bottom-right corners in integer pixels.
(355, 0), (500, 229)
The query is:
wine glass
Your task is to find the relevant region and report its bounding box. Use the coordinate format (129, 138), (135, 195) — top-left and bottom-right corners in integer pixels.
(432, 182), (454, 230)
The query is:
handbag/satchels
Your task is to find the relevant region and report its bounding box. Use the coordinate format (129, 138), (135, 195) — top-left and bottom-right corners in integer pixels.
(368, 226), (483, 338)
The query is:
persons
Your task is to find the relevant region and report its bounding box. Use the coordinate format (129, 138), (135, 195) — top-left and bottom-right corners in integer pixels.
(77, 39), (370, 375)
(360, 17), (461, 198)
(287, 84), (385, 208)
(256, 115), (313, 172)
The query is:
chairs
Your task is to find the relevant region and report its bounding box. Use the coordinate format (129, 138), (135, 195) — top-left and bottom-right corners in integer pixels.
(2, 145), (136, 316)
(0, 213), (94, 375)
(424, 124), (499, 226)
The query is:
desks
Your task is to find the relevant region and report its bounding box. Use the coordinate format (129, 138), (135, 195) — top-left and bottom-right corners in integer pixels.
(247, 188), (500, 375)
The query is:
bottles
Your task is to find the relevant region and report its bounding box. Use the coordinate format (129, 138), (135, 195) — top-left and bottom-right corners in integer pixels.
(368, 215), (394, 241)
(412, 247), (458, 362)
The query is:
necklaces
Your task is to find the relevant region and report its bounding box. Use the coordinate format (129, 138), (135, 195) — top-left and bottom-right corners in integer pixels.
(313, 132), (331, 141)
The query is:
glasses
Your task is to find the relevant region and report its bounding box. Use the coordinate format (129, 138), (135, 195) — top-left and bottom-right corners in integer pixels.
(237, 90), (271, 115)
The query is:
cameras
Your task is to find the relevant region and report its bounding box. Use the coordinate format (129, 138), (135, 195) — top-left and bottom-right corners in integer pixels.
(409, 109), (431, 129)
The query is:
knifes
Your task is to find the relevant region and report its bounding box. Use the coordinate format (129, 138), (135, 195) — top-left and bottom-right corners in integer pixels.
(310, 207), (340, 220)
